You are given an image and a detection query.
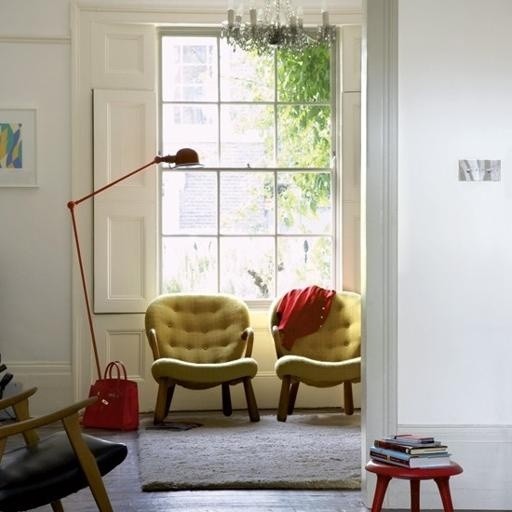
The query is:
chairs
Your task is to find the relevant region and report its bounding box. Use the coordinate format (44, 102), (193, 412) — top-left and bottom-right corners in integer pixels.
(145, 293), (262, 425)
(0, 387), (130, 512)
(269, 289), (361, 421)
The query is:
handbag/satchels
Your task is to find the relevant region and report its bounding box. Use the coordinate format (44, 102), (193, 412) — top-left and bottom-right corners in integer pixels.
(83, 360), (139, 431)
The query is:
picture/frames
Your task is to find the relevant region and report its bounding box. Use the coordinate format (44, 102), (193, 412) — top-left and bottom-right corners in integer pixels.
(0, 107), (40, 188)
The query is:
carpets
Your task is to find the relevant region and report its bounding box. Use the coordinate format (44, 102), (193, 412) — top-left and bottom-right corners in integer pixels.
(139, 411), (363, 492)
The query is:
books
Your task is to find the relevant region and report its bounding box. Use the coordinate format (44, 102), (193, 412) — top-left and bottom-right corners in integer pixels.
(369, 433), (453, 469)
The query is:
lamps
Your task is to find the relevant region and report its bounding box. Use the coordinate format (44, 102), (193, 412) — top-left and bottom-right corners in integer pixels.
(218, 0), (331, 57)
(67, 148), (204, 382)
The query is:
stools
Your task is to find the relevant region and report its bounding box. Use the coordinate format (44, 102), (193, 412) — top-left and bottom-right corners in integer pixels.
(365, 459), (463, 512)
